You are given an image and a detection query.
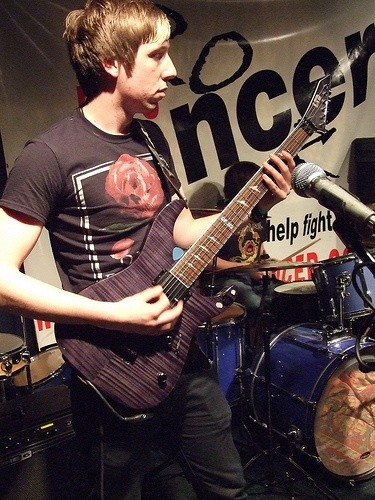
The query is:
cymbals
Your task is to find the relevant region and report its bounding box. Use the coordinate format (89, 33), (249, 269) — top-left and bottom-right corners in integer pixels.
(189, 207), (224, 218)
(205, 260), (322, 276)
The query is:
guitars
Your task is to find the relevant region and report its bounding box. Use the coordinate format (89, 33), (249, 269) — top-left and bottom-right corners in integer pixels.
(53, 74), (332, 411)
(0, 352), (27, 378)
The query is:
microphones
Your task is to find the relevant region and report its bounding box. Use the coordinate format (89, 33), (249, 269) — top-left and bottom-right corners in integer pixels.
(292, 162), (375, 230)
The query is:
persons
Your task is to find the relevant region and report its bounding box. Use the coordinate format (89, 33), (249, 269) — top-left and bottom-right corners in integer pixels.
(1, 0), (296, 500)
(199, 161), (287, 329)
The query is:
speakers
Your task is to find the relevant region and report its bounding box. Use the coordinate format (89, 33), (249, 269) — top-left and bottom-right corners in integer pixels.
(0, 416), (105, 500)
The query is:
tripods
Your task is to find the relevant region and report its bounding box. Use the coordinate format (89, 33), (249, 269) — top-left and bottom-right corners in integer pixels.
(240, 274), (339, 499)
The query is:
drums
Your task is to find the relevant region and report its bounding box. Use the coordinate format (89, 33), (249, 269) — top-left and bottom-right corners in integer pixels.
(247, 322), (375, 481)
(315, 253), (375, 321)
(10, 345), (72, 418)
(272, 280), (322, 322)
(194, 301), (247, 406)
(0, 332), (26, 359)
